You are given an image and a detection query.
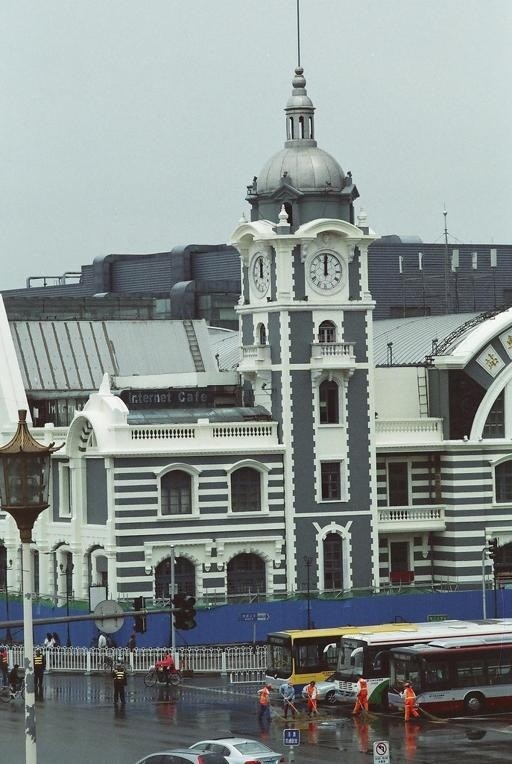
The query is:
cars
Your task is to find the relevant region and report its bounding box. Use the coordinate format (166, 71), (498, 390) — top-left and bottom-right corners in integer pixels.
(135, 736), (290, 764)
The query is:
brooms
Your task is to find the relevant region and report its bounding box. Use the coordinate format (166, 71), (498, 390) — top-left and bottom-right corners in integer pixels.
(357, 699), (378, 722)
(393, 687), (449, 722)
(267, 693), (329, 724)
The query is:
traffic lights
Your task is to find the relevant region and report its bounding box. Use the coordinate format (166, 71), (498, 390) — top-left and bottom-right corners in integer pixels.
(488, 538), (497, 560)
(132, 596), (147, 633)
(171, 592), (197, 630)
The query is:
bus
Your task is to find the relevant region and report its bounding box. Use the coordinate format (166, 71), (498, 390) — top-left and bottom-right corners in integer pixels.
(264, 617), (512, 718)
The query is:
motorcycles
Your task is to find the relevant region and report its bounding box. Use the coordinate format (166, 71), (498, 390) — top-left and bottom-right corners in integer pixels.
(144, 661), (182, 687)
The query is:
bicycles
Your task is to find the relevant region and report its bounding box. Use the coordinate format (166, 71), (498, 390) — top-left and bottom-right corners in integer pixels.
(0, 676), (25, 703)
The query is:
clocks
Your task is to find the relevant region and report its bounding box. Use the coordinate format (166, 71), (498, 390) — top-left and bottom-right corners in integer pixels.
(246, 249), (348, 300)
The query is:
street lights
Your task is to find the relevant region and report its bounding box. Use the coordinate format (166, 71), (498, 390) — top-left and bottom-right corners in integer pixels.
(303, 555), (314, 630)
(0, 409), (65, 764)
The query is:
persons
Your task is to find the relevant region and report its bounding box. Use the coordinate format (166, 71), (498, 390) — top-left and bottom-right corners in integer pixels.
(0, 647), (5, 669)
(279, 680), (295, 720)
(45, 631), (56, 650)
(52, 631), (61, 649)
(128, 629), (137, 651)
(304, 680), (318, 717)
(403, 721), (422, 763)
(97, 630), (106, 665)
(10, 663), (24, 699)
(257, 683), (273, 723)
(402, 682), (420, 721)
(34, 648), (45, 702)
(1, 646), (9, 685)
(104, 631), (115, 648)
(156, 653), (174, 688)
(111, 663), (129, 704)
(352, 673), (367, 717)
(351, 715), (369, 753)
